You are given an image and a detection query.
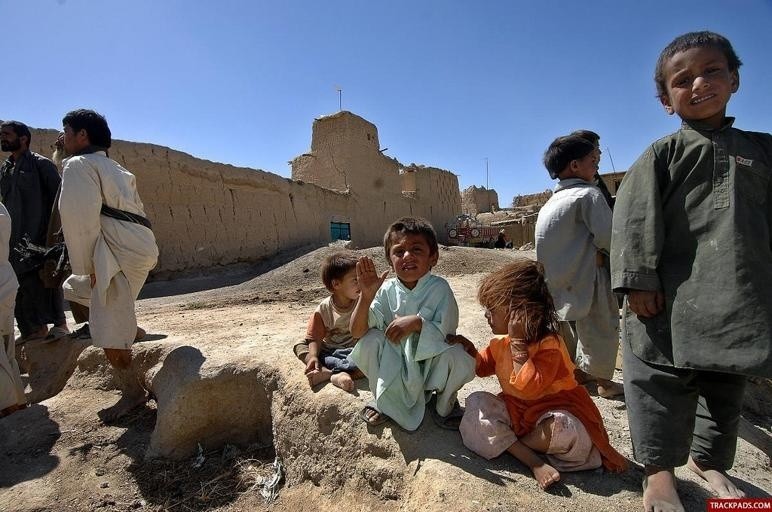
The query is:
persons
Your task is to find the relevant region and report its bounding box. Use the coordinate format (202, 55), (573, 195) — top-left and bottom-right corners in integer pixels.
(609, 30), (769, 511)
(456, 218), (514, 249)
(445, 259), (630, 489)
(2, 118), (62, 344)
(494, 228), (510, 250)
(2, 202), (29, 420)
(44, 122), (92, 339)
(536, 137), (624, 397)
(53, 107), (160, 426)
(294, 252), (363, 395)
(566, 128), (626, 321)
(347, 216), (475, 433)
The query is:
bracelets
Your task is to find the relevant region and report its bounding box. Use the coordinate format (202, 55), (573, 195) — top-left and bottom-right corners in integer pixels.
(509, 337), (529, 346)
(512, 350), (529, 363)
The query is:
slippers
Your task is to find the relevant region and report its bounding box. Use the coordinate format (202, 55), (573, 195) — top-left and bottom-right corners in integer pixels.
(358, 398), (389, 425)
(428, 393), (462, 430)
(15, 327), (70, 346)
